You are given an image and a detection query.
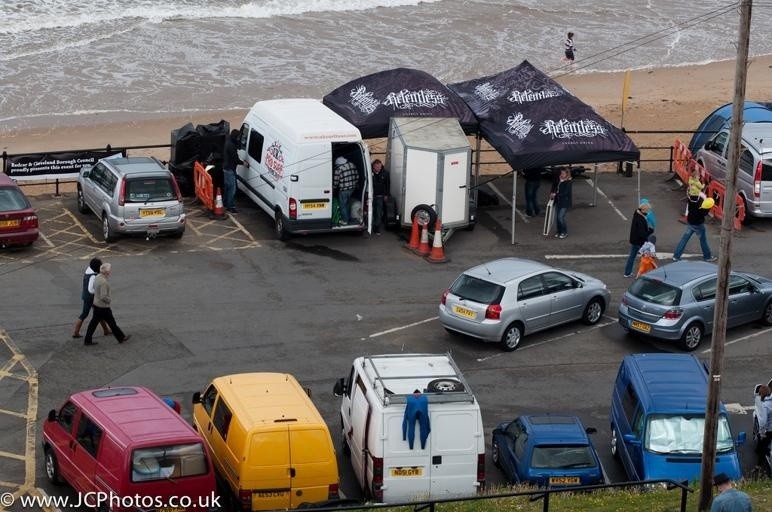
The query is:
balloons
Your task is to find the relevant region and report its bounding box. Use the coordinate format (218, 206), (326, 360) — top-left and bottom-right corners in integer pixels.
(701, 197), (715, 209)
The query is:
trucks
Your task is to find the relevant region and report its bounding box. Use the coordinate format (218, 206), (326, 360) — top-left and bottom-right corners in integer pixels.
(235, 99), (374, 239)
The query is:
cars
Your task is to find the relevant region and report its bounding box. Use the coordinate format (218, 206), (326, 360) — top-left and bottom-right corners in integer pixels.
(0, 170), (40, 250)
(752, 373), (772, 479)
(492, 414), (602, 493)
(439, 257), (611, 352)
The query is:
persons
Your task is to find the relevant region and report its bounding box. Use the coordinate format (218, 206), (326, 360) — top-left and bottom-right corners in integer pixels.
(634, 241), (657, 280)
(561, 32), (577, 73)
(711, 473), (752, 512)
(757, 386), (772, 480)
(333, 156), (359, 225)
(550, 167), (572, 238)
(222, 128), (248, 213)
(624, 204), (649, 278)
(371, 159), (390, 235)
(521, 167), (541, 218)
(84, 263), (132, 345)
(640, 199), (656, 244)
(673, 189), (717, 261)
(688, 171), (708, 200)
(72, 258), (113, 338)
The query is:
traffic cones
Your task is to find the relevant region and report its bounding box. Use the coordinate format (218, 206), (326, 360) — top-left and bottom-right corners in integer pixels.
(414, 218), (435, 258)
(424, 217), (451, 265)
(403, 216), (420, 252)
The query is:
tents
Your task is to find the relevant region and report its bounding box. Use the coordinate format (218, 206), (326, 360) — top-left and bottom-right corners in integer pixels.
(689, 101), (772, 157)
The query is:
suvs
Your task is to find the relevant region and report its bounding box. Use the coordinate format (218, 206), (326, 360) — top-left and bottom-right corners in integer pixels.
(618, 260), (772, 349)
(75, 155), (189, 241)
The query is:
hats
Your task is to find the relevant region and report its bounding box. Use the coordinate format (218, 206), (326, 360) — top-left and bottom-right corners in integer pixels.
(713, 473), (729, 486)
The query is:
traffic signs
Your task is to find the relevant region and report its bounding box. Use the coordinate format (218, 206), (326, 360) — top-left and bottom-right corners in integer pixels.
(209, 186), (228, 221)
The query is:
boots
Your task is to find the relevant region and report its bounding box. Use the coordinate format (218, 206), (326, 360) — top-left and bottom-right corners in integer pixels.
(100, 321), (112, 335)
(72, 319), (83, 337)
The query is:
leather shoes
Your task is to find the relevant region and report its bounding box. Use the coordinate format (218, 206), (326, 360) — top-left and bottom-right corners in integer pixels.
(85, 342), (98, 345)
(119, 335), (131, 343)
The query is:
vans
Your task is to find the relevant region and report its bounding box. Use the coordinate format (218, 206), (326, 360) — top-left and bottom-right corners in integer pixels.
(692, 121), (772, 220)
(608, 353), (742, 487)
(41, 385), (217, 511)
(192, 370), (341, 512)
(334, 351), (488, 506)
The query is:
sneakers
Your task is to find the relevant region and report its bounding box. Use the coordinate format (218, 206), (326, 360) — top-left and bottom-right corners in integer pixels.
(624, 272), (636, 277)
(226, 208), (239, 214)
(705, 256), (717, 262)
(555, 232), (568, 238)
